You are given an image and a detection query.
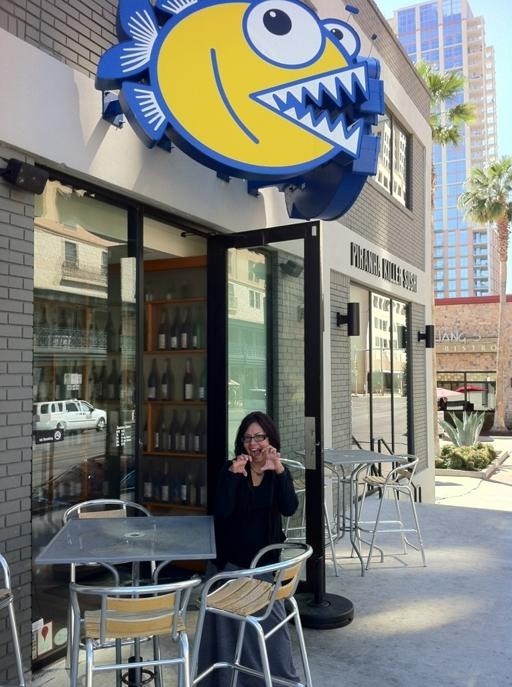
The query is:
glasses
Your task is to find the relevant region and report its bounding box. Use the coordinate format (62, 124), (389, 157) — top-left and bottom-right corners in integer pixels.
(241, 435), (267, 443)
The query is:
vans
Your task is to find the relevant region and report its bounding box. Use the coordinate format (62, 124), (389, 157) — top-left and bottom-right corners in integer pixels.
(32, 397), (107, 446)
(242, 389), (266, 416)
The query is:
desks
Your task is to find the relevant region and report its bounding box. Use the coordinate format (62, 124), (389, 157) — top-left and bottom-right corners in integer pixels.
(34, 517), (220, 687)
(295, 449), (405, 577)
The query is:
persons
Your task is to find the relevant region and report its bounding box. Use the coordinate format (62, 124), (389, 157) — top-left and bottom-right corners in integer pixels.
(437, 397), (447, 410)
(192, 411), (301, 687)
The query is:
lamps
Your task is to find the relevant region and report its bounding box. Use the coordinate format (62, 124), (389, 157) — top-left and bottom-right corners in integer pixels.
(337, 302), (360, 337)
(418, 325), (435, 349)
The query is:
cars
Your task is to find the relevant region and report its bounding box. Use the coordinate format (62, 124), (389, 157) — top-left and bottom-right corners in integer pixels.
(31, 452), (141, 588)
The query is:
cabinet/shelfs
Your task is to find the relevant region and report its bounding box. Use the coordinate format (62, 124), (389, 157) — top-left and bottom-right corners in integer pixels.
(143, 293), (207, 506)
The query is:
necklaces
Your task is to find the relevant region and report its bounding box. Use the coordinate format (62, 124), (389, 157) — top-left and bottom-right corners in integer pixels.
(250, 464), (264, 476)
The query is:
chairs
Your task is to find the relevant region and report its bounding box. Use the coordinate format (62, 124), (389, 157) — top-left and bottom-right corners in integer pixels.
(278, 458), (340, 582)
(64, 578), (206, 687)
(1, 553), (34, 686)
(60, 495), (170, 687)
(351, 454), (432, 573)
(191, 536), (317, 687)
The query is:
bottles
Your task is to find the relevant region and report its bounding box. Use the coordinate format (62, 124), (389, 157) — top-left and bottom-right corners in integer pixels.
(33, 307), (121, 354)
(147, 355), (206, 402)
(141, 458), (207, 509)
(142, 409), (204, 454)
(33, 462), (108, 502)
(157, 304), (193, 350)
(32, 358), (121, 403)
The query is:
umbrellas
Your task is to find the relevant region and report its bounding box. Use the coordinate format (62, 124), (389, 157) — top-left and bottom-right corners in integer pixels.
(436, 388), (462, 397)
(455, 383), (488, 401)
